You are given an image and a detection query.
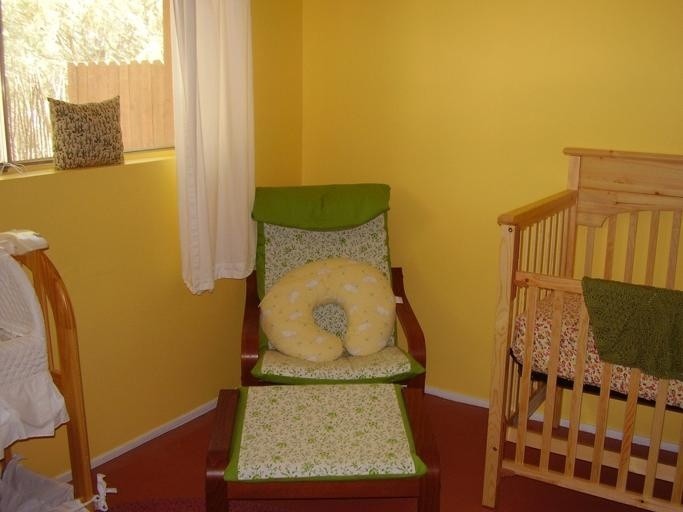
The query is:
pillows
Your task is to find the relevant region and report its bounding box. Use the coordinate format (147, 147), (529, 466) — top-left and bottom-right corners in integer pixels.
(252, 259), (398, 359)
(45, 94), (126, 168)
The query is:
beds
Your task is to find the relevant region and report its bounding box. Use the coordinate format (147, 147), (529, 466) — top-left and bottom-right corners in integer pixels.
(467, 147), (683, 512)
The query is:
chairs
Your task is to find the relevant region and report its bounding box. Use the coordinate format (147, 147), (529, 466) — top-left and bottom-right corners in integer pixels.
(231, 182), (428, 387)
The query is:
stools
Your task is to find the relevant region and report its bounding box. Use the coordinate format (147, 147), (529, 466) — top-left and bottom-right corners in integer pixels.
(201, 386), (442, 510)
